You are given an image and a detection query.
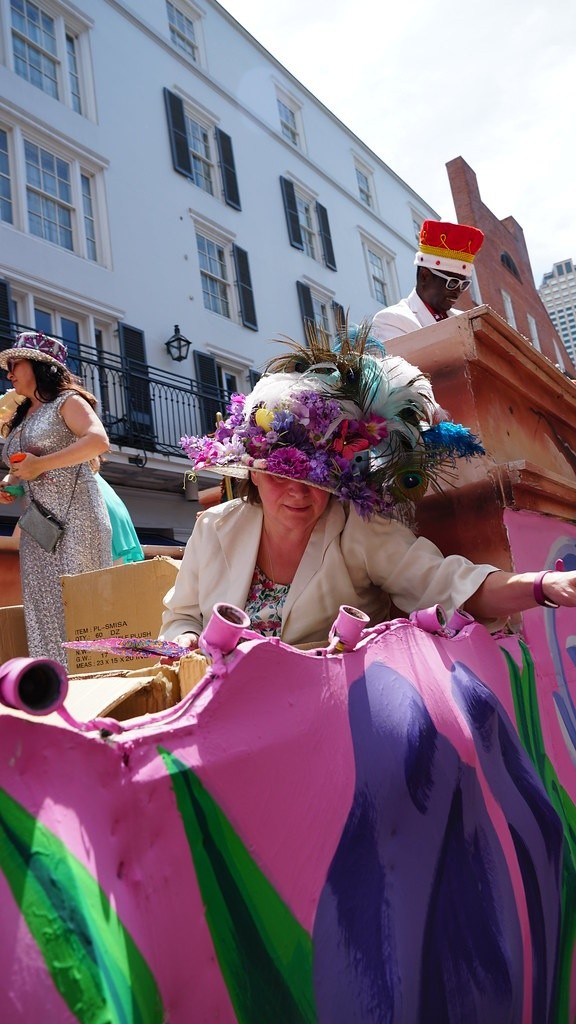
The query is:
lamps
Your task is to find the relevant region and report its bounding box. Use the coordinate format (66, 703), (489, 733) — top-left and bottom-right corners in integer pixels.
(165, 324), (192, 362)
(183, 478), (200, 502)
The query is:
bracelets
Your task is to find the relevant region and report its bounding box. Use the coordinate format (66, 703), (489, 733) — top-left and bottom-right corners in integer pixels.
(533, 559), (566, 609)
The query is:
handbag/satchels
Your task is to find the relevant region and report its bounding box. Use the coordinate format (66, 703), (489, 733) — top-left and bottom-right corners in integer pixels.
(17, 501), (63, 553)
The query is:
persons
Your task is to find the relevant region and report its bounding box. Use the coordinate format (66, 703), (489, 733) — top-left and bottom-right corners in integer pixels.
(0, 332), (113, 677)
(371, 219), (485, 342)
(0, 470), (145, 567)
(153, 371), (576, 654)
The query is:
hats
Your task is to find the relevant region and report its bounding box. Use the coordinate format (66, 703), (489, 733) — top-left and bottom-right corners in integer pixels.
(1, 329), (71, 380)
(196, 371), (372, 509)
(0, 387), (28, 443)
(415, 219), (484, 279)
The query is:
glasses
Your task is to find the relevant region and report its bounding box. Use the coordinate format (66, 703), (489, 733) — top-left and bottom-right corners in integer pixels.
(423, 266), (474, 292)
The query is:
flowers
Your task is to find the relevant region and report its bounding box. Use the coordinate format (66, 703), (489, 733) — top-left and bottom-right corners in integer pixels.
(175, 391), (393, 530)
